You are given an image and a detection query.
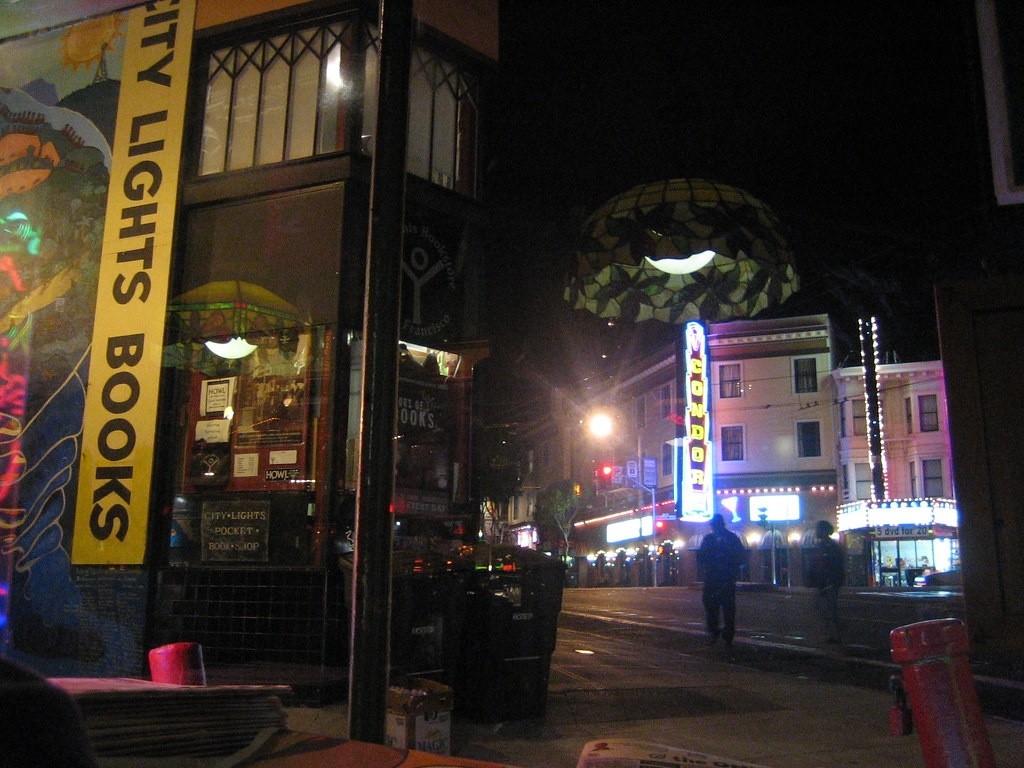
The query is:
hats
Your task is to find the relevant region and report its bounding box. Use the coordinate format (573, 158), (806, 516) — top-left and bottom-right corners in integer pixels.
(817, 520), (832, 534)
(708, 514), (724, 525)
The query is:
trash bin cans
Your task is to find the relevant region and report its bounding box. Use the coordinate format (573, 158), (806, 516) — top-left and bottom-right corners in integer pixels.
(439, 544), (568, 726)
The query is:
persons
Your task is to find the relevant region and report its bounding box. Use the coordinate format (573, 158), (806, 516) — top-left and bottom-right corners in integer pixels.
(808, 520), (844, 644)
(697, 514), (743, 647)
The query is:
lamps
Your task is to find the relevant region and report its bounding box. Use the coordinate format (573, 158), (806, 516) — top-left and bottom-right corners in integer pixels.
(162, 280), (325, 379)
(545, 175), (806, 326)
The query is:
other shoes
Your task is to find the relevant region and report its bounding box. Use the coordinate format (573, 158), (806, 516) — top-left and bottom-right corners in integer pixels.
(709, 628), (719, 643)
(722, 627), (732, 642)
(825, 635), (840, 645)
